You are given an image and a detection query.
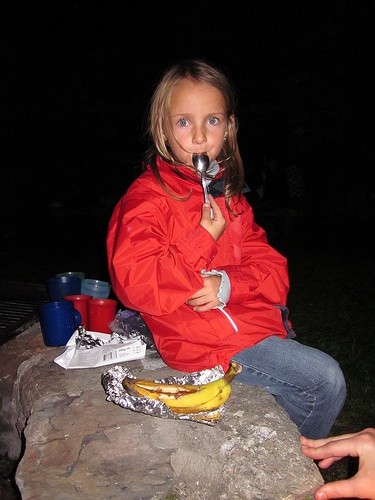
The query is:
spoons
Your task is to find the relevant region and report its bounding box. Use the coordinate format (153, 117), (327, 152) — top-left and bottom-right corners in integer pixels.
(192, 151), (214, 220)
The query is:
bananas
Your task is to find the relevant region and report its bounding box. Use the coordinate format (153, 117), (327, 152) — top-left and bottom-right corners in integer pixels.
(123, 362), (243, 414)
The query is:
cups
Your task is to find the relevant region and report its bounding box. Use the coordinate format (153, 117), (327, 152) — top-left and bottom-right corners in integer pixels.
(65, 295), (92, 331)
(41, 301), (81, 346)
(87, 299), (117, 334)
(48, 272), (84, 301)
(81, 280), (111, 299)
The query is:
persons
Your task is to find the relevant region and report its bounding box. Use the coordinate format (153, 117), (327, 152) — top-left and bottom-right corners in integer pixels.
(299, 425), (375, 500)
(105, 61), (348, 442)
